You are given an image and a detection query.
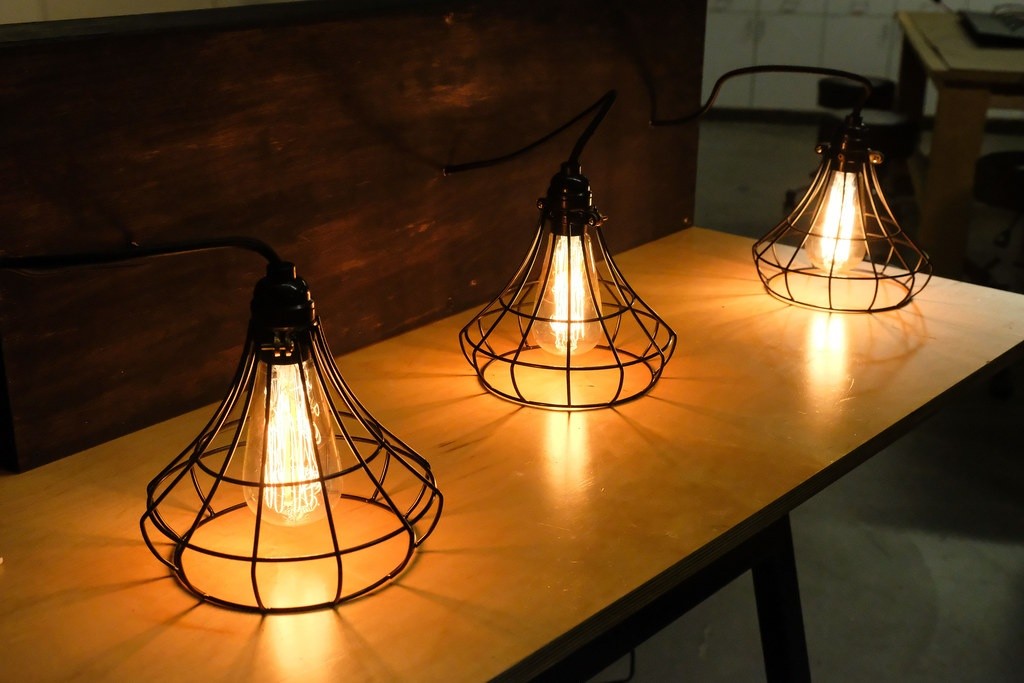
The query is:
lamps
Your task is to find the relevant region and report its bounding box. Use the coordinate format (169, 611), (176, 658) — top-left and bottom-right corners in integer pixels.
(442, 89), (677, 410)
(648, 63), (933, 312)
(0, 235), (444, 615)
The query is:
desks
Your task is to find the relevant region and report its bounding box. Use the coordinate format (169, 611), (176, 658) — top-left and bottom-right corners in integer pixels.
(0, 226), (1024, 683)
(894, 11), (1024, 278)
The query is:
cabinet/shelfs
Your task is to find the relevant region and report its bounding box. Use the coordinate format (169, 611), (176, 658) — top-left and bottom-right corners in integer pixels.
(700, 0), (1024, 125)
